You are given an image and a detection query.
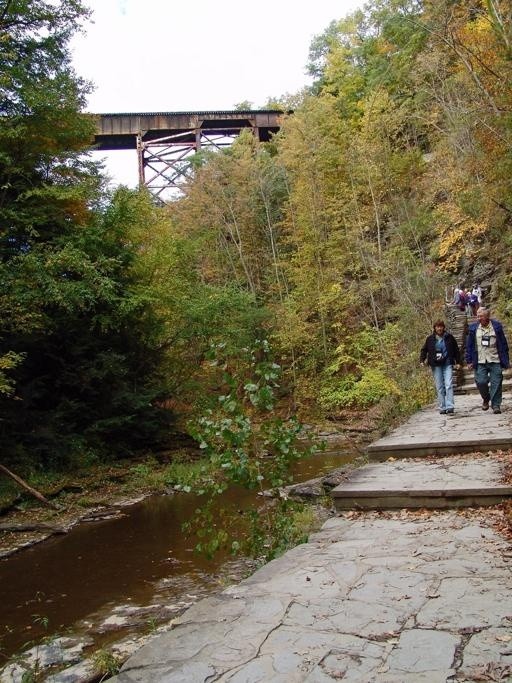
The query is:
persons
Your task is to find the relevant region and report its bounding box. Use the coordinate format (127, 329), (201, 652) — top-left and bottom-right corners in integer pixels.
(465, 307), (510, 415)
(449, 282), (483, 316)
(419, 318), (462, 415)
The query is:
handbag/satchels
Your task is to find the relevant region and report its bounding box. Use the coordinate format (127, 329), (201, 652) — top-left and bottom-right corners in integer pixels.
(435, 348), (446, 364)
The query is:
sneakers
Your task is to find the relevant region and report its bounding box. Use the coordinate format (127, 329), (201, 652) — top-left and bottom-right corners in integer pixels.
(439, 408), (454, 413)
(482, 393), (491, 410)
(492, 405), (501, 414)
